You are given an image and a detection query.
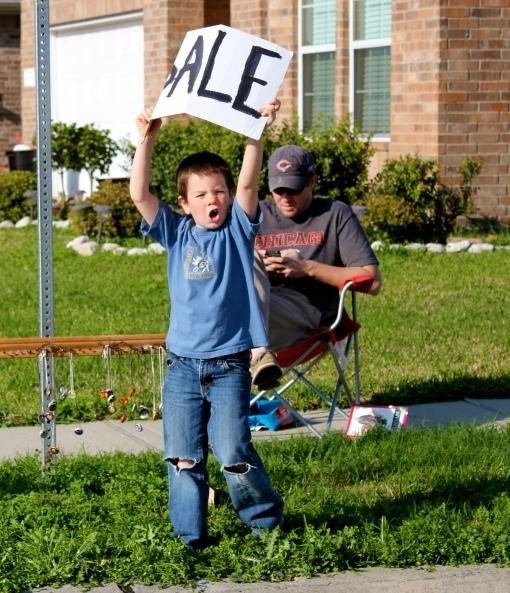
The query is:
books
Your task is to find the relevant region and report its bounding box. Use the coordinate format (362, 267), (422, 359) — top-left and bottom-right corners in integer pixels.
(345, 405), (408, 440)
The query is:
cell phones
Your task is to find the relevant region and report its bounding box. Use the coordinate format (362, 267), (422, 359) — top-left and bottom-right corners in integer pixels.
(265, 249), (281, 257)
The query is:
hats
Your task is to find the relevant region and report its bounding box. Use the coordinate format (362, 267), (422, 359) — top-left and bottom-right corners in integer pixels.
(268, 145), (315, 193)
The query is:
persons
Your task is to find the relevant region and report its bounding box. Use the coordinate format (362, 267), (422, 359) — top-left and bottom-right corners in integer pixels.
(248, 146), (381, 391)
(129, 98), (285, 548)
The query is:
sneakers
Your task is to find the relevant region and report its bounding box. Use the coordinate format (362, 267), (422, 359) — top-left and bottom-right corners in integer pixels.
(251, 354), (281, 391)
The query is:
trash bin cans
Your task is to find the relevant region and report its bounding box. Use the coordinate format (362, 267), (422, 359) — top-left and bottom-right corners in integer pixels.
(5, 150), (36, 172)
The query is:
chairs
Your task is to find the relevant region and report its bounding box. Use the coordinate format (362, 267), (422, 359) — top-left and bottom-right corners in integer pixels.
(248, 275), (382, 442)
